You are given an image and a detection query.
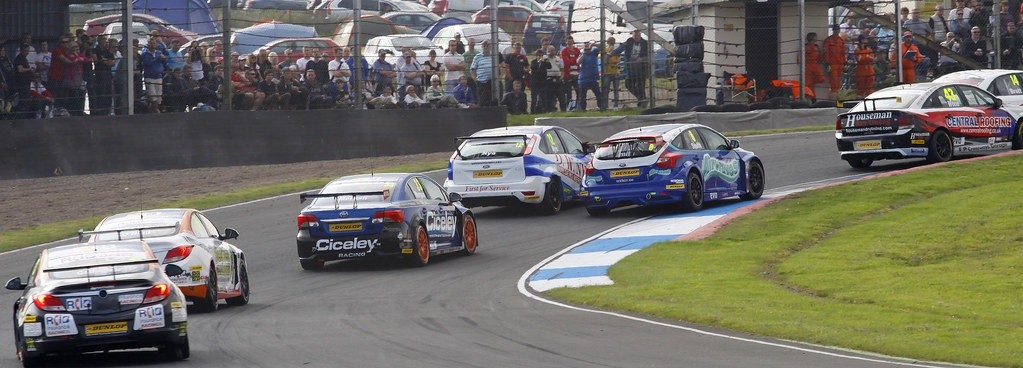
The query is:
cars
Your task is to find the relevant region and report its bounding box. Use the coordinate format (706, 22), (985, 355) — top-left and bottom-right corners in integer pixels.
(296, 148), (480, 272)
(66, 0), (575, 74)
(836, 63), (1023, 170)
(443, 124), (766, 218)
(6, 209), (250, 368)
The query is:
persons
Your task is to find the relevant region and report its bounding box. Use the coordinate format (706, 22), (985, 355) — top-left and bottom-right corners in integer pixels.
(837, 0), (1023, 82)
(797, 32), (826, 103)
(823, 24), (846, 101)
(606, 30), (648, 108)
(854, 34), (875, 100)
(890, 31), (922, 83)
(0, 32), (619, 119)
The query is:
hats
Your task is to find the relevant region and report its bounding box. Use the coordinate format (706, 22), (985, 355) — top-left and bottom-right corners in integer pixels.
(377, 49), (387, 55)
(858, 34), (869, 40)
(404, 51), (411, 59)
(832, 24), (839, 29)
(147, 30), (159, 36)
(905, 31), (912, 38)
(454, 33), (460, 39)
(237, 55), (247, 61)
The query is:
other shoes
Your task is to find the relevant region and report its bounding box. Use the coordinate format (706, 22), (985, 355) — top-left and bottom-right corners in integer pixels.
(457, 103), (470, 109)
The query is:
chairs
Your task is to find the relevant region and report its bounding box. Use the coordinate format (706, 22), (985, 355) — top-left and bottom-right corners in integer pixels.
(722, 70), (758, 103)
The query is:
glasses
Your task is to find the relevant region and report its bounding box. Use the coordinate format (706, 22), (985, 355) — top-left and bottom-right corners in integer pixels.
(956, 12), (963, 15)
(61, 41), (70, 43)
(947, 36), (955, 39)
(972, 31), (981, 34)
